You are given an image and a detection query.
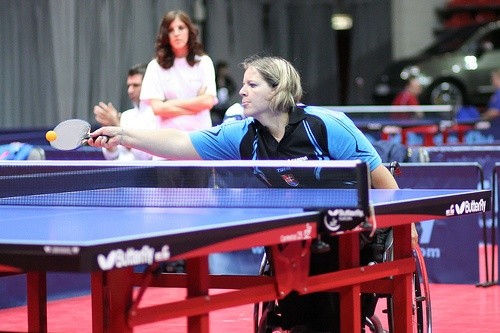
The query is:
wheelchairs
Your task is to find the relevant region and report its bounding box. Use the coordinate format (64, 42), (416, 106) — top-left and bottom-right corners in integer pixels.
(253, 160), (432, 333)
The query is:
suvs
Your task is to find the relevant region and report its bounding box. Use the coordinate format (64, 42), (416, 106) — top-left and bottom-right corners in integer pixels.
(373, 19), (500, 119)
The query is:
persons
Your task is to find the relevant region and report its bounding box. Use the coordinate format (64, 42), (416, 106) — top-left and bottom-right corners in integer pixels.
(138, 11), (219, 161)
(85, 56), (418, 333)
(93, 62), (155, 161)
(210, 61), (236, 126)
(392, 78), (424, 120)
(480, 69), (500, 139)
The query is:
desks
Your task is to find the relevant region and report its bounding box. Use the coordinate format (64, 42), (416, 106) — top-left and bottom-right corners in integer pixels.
(0, 157), (495, 333)
(332, 105), (456, 138)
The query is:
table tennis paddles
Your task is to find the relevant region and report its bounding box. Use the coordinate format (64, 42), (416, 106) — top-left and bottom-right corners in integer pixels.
(49, 118), (109, 151)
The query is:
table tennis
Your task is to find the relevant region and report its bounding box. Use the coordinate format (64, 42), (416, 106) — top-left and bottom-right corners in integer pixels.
(46, 131), (57, 141)
